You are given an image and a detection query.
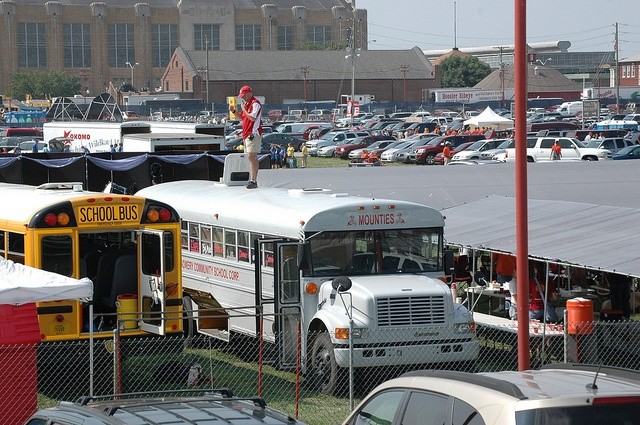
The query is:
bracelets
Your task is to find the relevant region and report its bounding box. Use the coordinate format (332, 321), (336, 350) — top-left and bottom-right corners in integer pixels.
(232, 110), (236, 114)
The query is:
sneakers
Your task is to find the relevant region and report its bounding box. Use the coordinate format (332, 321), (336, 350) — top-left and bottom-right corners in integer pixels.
(246, 180), (257, 188)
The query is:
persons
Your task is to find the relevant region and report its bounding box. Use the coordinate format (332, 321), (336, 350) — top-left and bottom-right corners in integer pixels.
(111, 144), (118, 153)
(118, 142), (123, 152)
(287, 143), (295, 167)
(404, 127), (418, 138)
(509, 267), (517, 320)
(623, 127), (635, 139)
(229, 86), (263, 189)
(442, 140), (452, 165)
(372, 128), (390, 137)
(438, 128), (442, 136)
(301, 142), (308, 168)
(81, 146), (90, 157)
(423, 127), (430, 133)
(110, 144), (116, 153)
(234, 130), (240, 138)
(270, 143), (287, 168)
(492, 252), (516, 312)
(348, 122), (352, 128)
(447, 126), (493, 136)
(224, 141), (244, 150)
(32, 140), (39, 153)
(358, 122), (363, 130)
(309, 129), (318, 140)
(13, 142), (22, 153)
(434, 126), (438, 133)
(42, 144), (48, 152)
(584, 131), (605, 140)
(361, 147), (382, 164)
(550, 141), (562, 160)
(528, 261), (558, 322)
(64, 144), (71, 153)
(606, 272), (634, 320)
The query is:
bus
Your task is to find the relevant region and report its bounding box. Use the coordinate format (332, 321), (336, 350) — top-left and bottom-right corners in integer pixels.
(0, 180), (185, 362)
(134, 178), (480, 396)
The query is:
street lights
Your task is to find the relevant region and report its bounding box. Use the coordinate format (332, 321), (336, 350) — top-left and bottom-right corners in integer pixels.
(125, 61), (140, 86)
(399, 62), (411, 102)
(299, 64), (311, 111)
(536, 57), (551, 66)
(196, 61), (210, 106)
(342, 53), (360, 95)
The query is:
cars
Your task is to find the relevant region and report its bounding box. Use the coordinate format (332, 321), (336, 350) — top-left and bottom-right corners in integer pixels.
(122, 110), (140, 120)
(226, 98), (640, 165)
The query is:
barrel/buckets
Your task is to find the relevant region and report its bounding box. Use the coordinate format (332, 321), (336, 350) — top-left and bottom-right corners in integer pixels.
(566, 299), (595, 335)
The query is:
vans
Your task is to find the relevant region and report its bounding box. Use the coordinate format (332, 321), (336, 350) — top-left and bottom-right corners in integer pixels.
(196, 109), (218, 118)
(152, 111), (168, 117)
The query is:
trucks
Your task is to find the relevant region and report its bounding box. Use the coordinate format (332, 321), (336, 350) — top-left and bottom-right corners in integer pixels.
(42, 121), (151, 153)
(147, 121), (225, 135)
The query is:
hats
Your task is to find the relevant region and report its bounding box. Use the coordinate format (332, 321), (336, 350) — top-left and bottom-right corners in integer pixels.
(237, 86), (252, 98)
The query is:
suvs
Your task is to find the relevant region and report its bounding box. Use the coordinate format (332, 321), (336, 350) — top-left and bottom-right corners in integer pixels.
(339, 363), (640, 424)
(0, 127), (43, 153)
(22, 388), (308, 425)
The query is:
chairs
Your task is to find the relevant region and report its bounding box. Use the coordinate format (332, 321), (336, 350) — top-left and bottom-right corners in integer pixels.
(84, 251), (104, 271)
(101, 255), (138, 307)
(92, 252), (128, 304)
(453, 255), (472, 294)
(481, 255), (491, 276)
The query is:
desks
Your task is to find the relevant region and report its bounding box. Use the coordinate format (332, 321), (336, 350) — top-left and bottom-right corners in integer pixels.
(464, 284), (511, 315)
